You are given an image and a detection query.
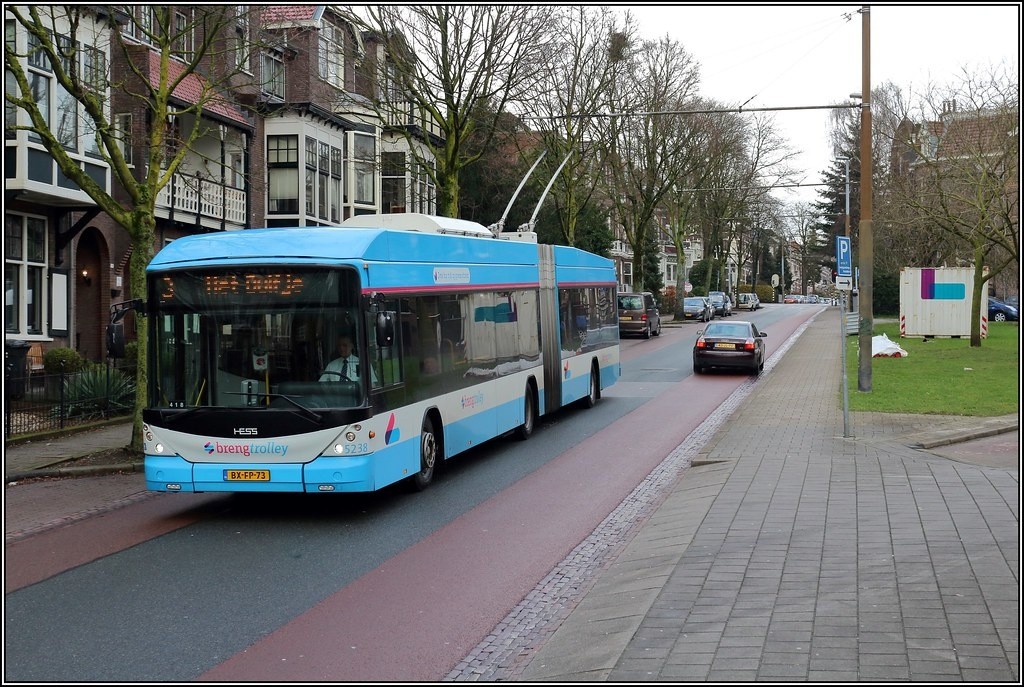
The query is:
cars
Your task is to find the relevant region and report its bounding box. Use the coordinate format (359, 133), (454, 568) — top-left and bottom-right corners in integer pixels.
(724, 295), (732, 316)
(693, 320), (768, 375)
(683, 296), (715, 322)
(988, 297), (1018, 323)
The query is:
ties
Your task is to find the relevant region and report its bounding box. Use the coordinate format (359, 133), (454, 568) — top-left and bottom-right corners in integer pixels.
(340, 359), (348, 381)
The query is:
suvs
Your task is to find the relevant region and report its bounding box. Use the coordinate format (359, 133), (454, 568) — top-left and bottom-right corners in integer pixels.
(709, 292), (728, 317)
(738, 293), (760, 311)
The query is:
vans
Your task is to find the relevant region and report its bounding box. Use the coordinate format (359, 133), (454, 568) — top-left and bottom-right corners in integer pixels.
(618, 292), (661, 339)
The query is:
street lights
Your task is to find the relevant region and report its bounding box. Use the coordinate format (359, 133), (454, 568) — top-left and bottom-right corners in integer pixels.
(837, 157), (851, 312)
(784, 294), (833, 304)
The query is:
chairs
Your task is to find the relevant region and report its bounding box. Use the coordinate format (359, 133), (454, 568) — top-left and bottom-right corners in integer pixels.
(331, 299), (588, 381)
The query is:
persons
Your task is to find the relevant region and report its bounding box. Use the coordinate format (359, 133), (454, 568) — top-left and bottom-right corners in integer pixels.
(424, 357), (439, 376)
(319, 335), (380, 389)
(289, 323), (329, 382)
(226, 326), (280, 405)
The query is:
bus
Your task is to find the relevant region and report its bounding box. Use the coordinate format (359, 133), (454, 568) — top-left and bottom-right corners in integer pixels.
(106, 213), (621, 492)
